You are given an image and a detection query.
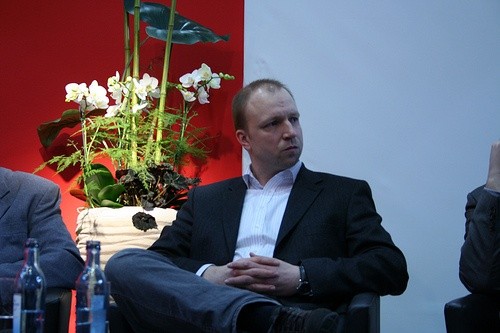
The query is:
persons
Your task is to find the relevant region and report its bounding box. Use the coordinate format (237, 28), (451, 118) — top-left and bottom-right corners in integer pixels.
(0, 167), (84, 307)
(459, 143), (500, 293)
(103, 79), (409, 333)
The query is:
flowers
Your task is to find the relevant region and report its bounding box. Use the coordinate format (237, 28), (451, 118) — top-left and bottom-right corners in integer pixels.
(37, 0), (235, 207)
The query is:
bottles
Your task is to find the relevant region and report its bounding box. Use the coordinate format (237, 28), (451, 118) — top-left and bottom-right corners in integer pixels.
(76, 240), (110, 333)
(12, 238), (45, 333)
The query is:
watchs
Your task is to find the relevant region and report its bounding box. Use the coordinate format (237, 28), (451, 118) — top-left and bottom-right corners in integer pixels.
(296, 265), (311, 296)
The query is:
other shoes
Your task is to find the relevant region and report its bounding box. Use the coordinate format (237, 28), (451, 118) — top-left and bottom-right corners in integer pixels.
(273, 307), (339, 332)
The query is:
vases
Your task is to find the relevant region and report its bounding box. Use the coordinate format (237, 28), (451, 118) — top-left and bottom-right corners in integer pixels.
(76, 205), (179, 307)
